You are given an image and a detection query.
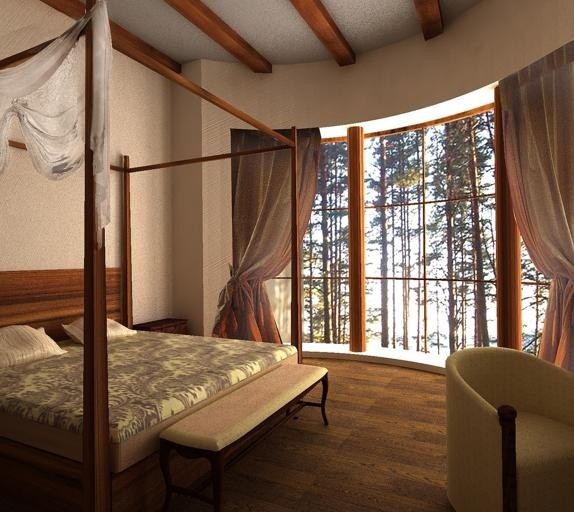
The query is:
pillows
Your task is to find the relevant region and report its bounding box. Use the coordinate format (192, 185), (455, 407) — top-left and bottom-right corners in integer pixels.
(61, 317), (138, 344)
(0, 325), (69, 367)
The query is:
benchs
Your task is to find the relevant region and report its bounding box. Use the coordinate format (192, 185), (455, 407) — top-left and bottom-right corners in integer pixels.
(157, 362), (329, 512)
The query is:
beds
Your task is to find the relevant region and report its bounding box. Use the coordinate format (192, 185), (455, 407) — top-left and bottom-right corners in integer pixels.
(0, 1), (298, 512)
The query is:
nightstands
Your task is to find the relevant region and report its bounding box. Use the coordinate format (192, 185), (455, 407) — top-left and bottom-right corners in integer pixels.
(132, 318), (191, 335)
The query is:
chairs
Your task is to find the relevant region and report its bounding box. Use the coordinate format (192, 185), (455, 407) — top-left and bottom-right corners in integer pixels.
(446, 346), (574, 512)
